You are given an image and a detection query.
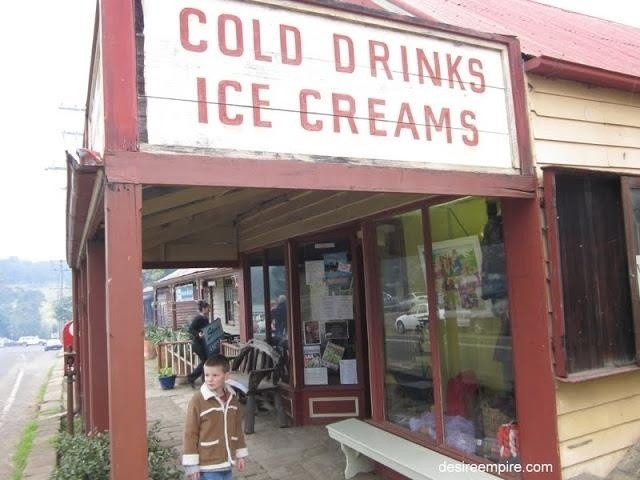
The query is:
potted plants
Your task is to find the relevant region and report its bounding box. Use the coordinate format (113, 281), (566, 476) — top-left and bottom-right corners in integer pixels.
(159, 367), (177, 389)
(143, 323), (158, 359)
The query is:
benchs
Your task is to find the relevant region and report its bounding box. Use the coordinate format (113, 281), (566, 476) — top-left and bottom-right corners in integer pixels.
(326, 416), (505, 480)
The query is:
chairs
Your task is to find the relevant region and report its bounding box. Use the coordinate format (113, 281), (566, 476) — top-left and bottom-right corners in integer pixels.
(226, 337), (292, 434)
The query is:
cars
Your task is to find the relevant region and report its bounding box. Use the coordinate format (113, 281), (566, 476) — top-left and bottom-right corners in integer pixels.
(18, 336), (62, 351)
(395, 291), (445, 334)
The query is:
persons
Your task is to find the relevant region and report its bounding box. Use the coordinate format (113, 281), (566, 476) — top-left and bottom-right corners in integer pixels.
(181, 353), (249, 480)
(304, 321), (318, 344)
(271, 294), (286, 338)
(186, 301), (211, 390)
